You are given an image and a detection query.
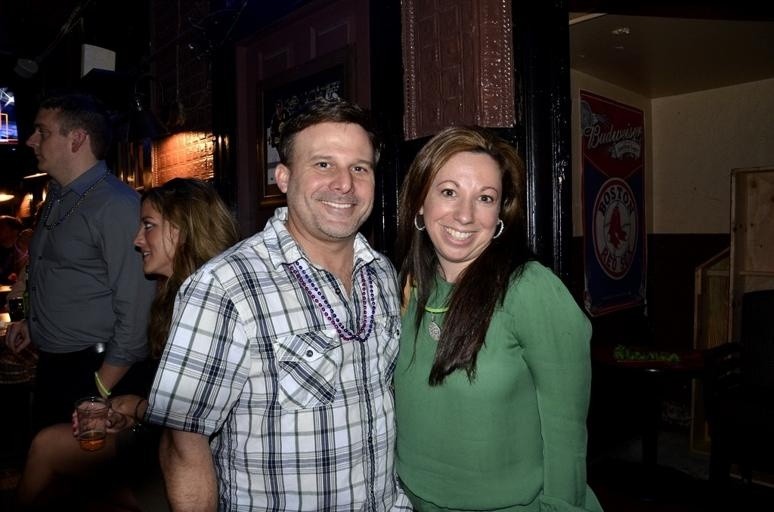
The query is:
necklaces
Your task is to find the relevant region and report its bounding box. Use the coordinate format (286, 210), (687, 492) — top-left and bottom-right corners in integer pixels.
(289, 262), (367, 341)
(41, 171), (111, 230)
(294, 260), (375, 343)
(412, 280), (448, 340)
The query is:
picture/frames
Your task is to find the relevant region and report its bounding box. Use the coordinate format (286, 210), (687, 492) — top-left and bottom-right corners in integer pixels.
(256, 43), (357, 210)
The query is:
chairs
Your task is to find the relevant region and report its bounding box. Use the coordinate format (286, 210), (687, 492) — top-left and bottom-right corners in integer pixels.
(702, 341), (772, 510)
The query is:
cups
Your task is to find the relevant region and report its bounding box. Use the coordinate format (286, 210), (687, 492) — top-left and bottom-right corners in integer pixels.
(75, 395), (110, 452)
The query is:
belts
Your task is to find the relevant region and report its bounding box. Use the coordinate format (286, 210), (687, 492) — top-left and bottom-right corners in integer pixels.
(37, 343), (106, 366)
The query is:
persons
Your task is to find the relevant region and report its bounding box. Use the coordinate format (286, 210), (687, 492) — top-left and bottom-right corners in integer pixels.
(15, 175), (244, 512)
(0, 215), (22, 286)
(135, 90), (416, 512)
(15, 201), (44, 267)
(0, 89), (155, 414)
(386, 127), (606, 511)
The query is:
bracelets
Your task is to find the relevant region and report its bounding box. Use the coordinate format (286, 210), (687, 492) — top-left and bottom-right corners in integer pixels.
(94, 371), (111, 395)
(132, 397), (146, 419)
